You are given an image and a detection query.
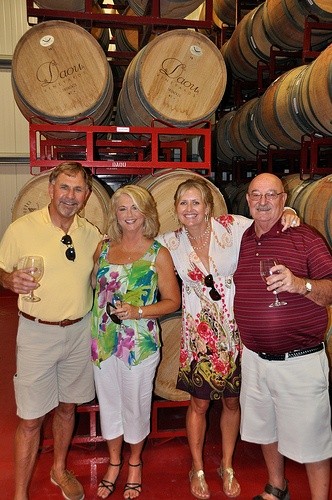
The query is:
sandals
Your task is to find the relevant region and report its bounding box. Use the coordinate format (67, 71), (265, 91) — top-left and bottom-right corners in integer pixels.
(189, 468), (209, 499)
(98, 457), (122, 497)
(216, 458), (240, 497)
(250, 479), (289, 500)
(123, 459), (143, 499)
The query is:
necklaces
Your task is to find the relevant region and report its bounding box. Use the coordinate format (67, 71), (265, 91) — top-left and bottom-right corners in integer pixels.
(185, 221), (210, 249)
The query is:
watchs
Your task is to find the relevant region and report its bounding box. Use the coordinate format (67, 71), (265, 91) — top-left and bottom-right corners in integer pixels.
(304, 279), (312, 295)
(139, 307), (143, 318)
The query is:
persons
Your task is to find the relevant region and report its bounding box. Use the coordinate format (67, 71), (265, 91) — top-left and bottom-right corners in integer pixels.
(0, 161), (110, 500)
(91, 185), (181, 499)
(153, 179), (300, 499)
(234, 173), (332, 500)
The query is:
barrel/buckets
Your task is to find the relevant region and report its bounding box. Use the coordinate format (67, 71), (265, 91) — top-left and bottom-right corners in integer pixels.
(11, 1), (332, 402)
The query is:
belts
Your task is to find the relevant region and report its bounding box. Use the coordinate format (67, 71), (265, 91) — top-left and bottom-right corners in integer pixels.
(21, 312), (84, 327)
(253, 343), (325, 362)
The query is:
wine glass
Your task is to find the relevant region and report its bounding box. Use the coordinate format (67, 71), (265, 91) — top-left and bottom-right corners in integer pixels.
(21, 256), (45, 302)
(260, 257), (287, 307)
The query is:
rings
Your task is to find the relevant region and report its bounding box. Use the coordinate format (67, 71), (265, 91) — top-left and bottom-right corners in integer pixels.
(126, 311), (128, 316)
(293, 221), (296, 224)
(282, 280), (283, 286)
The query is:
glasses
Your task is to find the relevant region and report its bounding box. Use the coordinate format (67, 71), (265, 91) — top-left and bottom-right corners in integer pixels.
(248, 191), (284, 200)
(62, 233), (76, 262)
(204, 274), (220, 301)
(106, 302), (122, 325)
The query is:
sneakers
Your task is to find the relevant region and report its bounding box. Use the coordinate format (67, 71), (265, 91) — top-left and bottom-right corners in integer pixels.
(50, 468), (84, 500)
